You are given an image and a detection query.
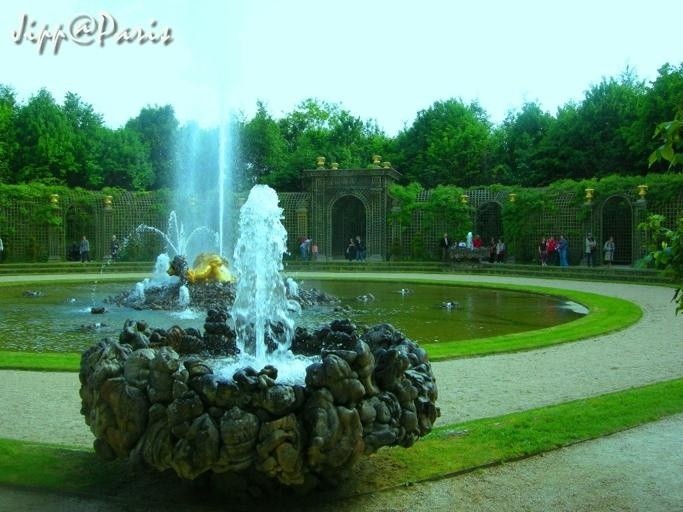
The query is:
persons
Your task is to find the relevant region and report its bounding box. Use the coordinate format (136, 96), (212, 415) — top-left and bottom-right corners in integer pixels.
(80, 235), (91, 264)
(538, 234), (571, 267)
(439, 232), (450, 260)
(583, 232), (597, 267)
(345, 235), (368, 260)
(299, 236), (318, 260)
(110, 234), (120, 261)
(466, 228), (505, 262)
(603, 235), (616, 266)
